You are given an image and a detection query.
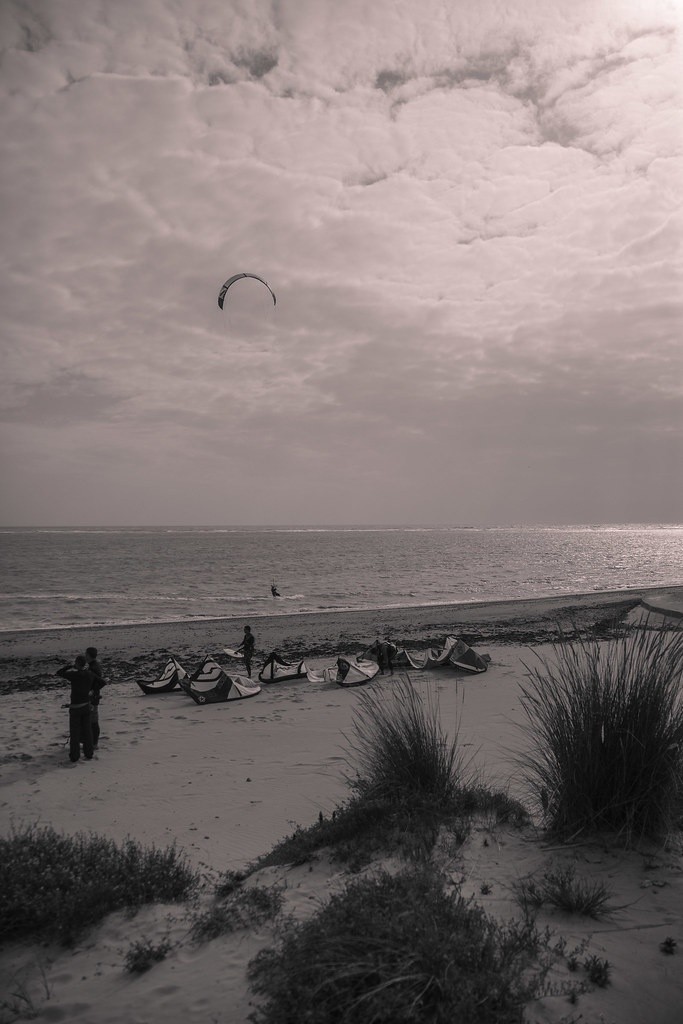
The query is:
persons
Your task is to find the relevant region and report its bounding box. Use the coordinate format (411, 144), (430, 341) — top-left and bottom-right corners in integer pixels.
(57, 655), (106, 762)
(85, 647), (102, 748)
(271, 586), (277, 597)
(238, 625), (255, 677)
